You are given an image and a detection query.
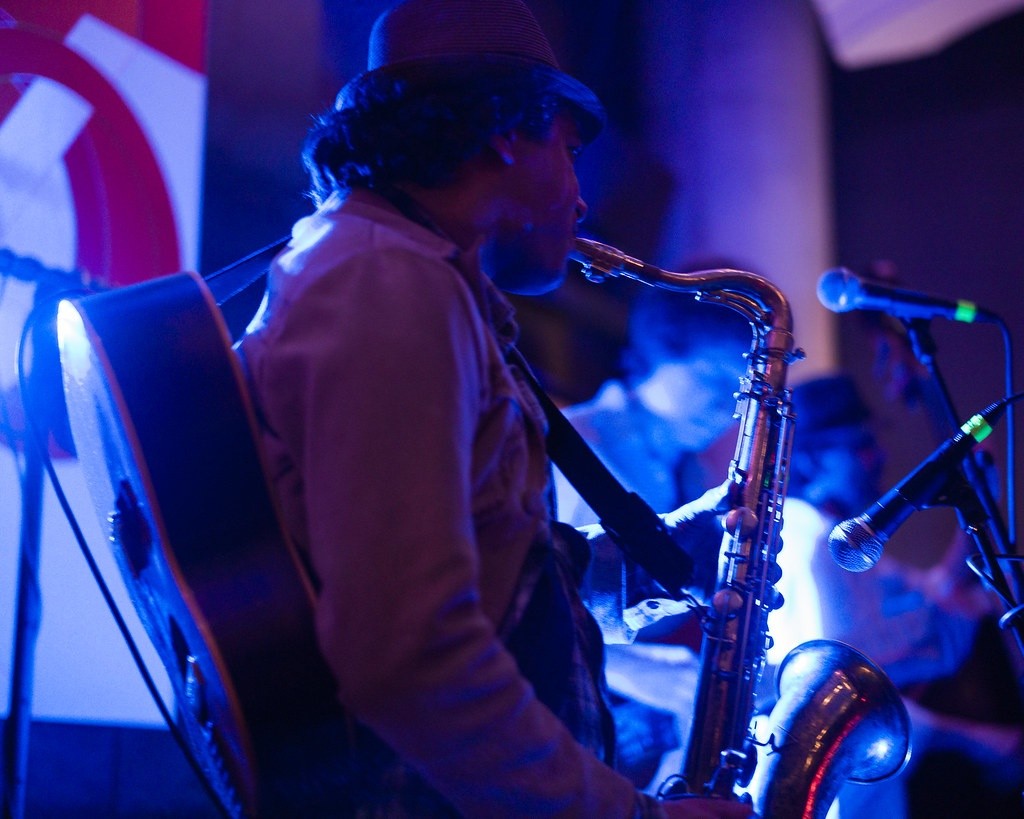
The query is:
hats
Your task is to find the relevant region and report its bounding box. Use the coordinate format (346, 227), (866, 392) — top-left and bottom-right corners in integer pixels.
(793, 376), (871, 432)
(335, 0), (606, 142)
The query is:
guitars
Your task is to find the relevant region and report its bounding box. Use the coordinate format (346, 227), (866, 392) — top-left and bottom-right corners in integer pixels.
(56, 268), (360, 819)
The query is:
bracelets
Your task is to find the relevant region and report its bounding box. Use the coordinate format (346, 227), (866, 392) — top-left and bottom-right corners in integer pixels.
(634, 793), (661, 819)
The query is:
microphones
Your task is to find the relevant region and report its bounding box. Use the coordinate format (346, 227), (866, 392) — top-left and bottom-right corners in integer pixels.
(815, 266), (990, 328)
(828, 399), (1006, 573)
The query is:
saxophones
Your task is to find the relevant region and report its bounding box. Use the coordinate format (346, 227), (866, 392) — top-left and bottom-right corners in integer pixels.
(568, 238), (912, 819)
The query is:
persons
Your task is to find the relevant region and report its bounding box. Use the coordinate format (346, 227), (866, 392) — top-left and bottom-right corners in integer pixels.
(232, 2), (754, 819)
(552, 260), (989, 819)
